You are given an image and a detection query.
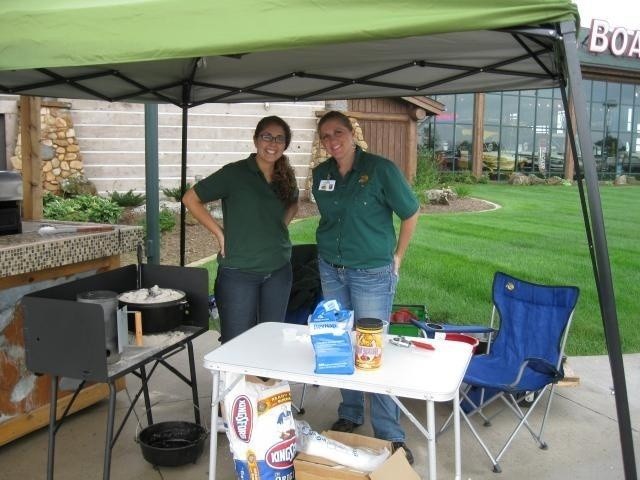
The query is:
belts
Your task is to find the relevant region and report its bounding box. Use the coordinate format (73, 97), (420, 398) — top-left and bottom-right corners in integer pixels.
(325, 260), (347, 269)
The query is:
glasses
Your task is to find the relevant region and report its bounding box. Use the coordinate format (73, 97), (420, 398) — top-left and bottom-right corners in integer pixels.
(259, 134), (288, 144)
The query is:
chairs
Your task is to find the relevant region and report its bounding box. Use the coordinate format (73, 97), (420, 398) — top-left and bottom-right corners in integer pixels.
(435, 271), (580, 472)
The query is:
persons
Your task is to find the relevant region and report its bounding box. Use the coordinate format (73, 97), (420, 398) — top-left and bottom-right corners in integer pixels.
(310, 111), (423, 466)
(181, 115), (299, 432)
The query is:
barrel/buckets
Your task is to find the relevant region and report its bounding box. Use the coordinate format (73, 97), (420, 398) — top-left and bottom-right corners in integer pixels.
(132, 399), (210, 469)
(446, 334), (479, 352)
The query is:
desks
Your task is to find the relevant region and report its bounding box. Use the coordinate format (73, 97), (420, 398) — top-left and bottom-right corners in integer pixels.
(204, 321), (473, 480)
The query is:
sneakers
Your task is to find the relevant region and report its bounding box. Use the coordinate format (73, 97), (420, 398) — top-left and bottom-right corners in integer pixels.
(331, 417), (360, 432)
(390, 440), (414, 467)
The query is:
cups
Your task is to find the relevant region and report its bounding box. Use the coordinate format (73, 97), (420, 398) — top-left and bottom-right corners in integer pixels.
(382, 319), (389, 346)
